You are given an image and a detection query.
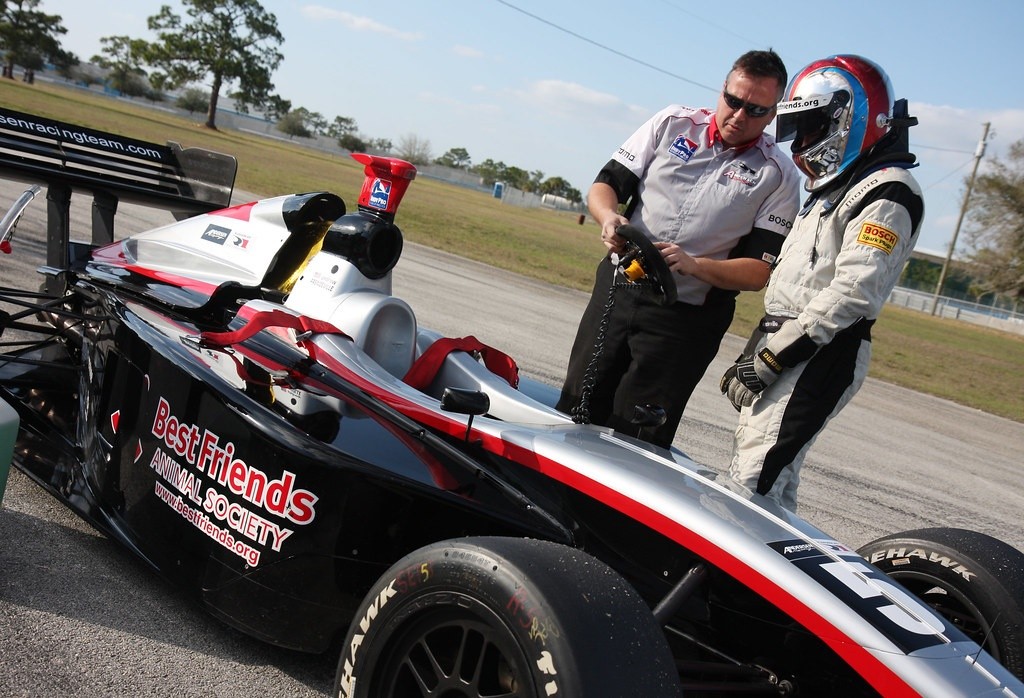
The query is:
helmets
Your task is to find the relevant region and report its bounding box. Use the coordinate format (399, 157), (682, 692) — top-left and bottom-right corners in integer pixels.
(776, 55), (895, 192)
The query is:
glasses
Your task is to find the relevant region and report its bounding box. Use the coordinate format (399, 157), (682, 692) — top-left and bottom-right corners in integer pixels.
(723, 83), (779, 118)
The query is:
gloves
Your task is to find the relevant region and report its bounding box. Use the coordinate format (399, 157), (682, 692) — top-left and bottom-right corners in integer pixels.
(721, 314), (823, 407)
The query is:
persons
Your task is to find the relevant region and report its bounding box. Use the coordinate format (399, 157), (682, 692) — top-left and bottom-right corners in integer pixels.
(721, 54), (924, 516)
(554, 48), (800, 451)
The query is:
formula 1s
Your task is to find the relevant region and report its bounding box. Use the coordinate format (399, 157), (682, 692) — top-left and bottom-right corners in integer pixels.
(0, 106), (1023, 698)
